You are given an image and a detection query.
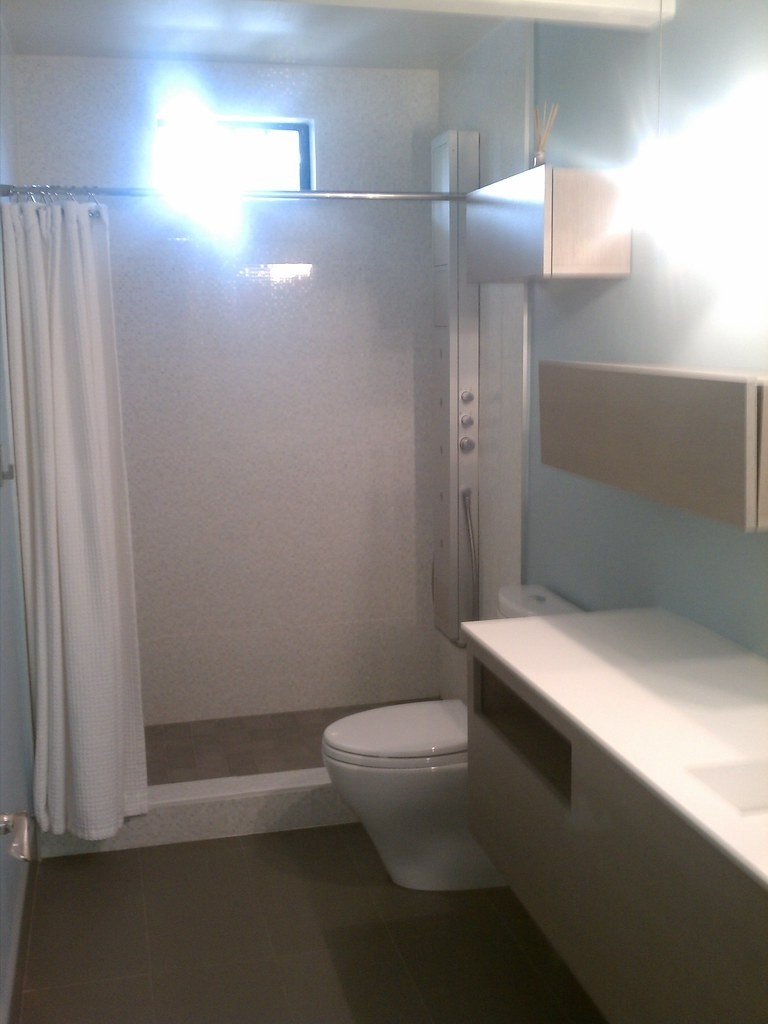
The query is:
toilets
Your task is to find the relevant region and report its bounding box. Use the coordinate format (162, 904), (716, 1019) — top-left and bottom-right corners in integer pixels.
(321, 582), (590, 893)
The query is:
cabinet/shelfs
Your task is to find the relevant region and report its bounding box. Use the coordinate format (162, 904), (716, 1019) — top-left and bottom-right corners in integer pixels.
(461, 603), (768, 1024)
(467, 163), (630, 282)
